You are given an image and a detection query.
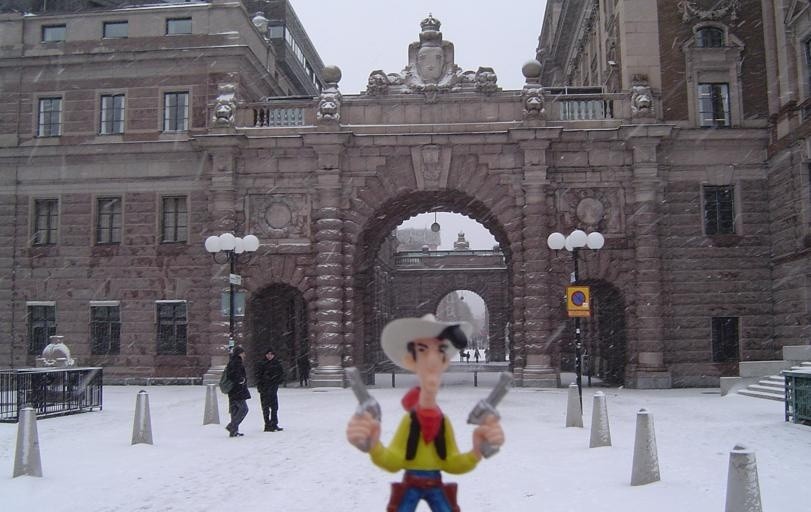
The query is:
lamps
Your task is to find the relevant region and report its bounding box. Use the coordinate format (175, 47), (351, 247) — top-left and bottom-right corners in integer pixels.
(430, 210), (441, 233)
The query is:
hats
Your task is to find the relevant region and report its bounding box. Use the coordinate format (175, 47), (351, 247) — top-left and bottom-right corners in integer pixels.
(233, 346), (246, 356)
(264, 345), (276, 354)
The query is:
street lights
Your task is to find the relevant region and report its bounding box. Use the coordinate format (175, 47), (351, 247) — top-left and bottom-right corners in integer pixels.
(545, 230), (604, 413)
(205, 232), (259, 357)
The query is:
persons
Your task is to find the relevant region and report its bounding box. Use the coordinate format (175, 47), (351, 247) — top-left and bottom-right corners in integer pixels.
(257, 346), (283, 432)
(224, 347), (251, 437)
(458, 348), (490, 362)
(344, 313), (513, 512)
(296, 352), (309, 387)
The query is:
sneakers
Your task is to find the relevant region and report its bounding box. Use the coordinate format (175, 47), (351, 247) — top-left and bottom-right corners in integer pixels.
(225, 425), (244, 438)
(264, 425), (284, 432)
